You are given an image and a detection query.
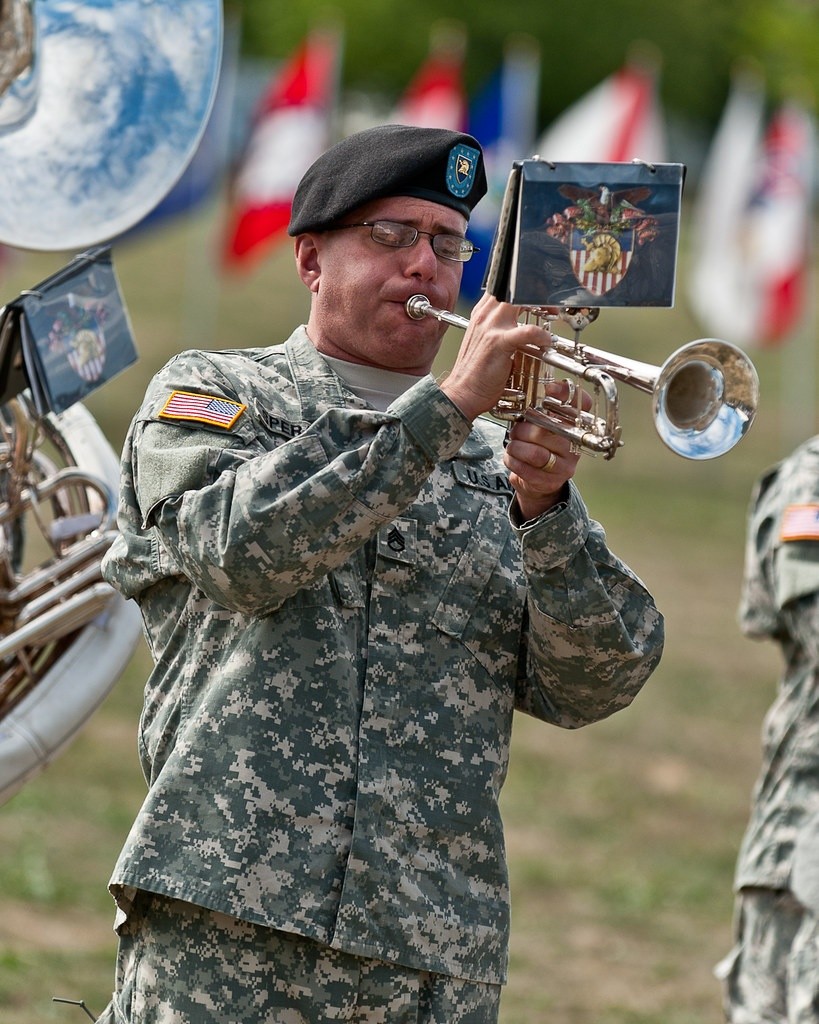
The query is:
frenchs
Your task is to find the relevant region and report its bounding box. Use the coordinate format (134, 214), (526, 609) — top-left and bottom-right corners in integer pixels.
(0, 0), (225, 792)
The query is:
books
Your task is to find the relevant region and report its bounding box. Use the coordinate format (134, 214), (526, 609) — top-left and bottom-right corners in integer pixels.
(481, 153), (689, 310)
(0, 246), (142, 423)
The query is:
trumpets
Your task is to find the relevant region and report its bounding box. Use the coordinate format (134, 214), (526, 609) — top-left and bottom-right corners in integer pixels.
(404, 295), (760, 464)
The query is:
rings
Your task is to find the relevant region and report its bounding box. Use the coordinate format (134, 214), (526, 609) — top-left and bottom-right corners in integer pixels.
(542, 450), (558, 474)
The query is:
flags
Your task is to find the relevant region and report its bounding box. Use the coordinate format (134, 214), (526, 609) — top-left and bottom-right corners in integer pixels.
(114, 9), (819, 364)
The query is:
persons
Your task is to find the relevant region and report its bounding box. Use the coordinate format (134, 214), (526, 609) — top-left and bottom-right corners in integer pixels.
(81, 124), (669, 1024)
(708, 411), (819, 1024)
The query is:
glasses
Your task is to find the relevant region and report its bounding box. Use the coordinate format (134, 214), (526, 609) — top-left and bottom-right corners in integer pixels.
(322, 220), (481, 262)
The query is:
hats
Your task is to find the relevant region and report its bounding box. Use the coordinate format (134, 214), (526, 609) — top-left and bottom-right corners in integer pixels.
(287, 124), (488, 239)
